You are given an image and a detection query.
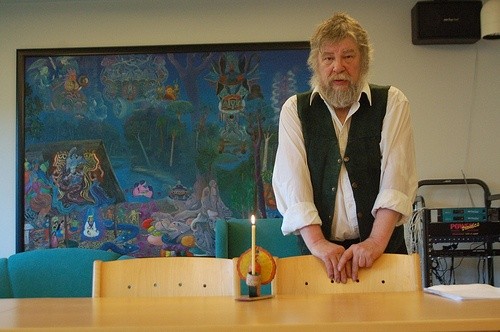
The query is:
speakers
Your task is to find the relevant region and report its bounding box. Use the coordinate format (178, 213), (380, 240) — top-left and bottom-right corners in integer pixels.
(411, 0), (482, 44)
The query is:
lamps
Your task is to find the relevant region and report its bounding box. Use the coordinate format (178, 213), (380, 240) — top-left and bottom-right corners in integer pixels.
(480, 0), (500, 42)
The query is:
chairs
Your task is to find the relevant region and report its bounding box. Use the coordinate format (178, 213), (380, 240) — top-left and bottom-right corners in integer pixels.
(270, 253), (423, 295)
(91, 257), (242, 297)
(215, 218), (301, 296)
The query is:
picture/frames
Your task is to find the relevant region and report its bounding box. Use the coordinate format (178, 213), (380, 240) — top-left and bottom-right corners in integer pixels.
(14, 40), (313, 258)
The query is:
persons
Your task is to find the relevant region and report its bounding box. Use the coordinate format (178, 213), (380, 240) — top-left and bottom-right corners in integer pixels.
(272, 12), (418, 284)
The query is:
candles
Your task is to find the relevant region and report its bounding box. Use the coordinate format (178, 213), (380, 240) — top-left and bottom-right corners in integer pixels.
(250, 214), (256, 275)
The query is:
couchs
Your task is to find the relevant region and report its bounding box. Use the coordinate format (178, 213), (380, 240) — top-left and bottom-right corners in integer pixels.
(0, 248), (135, 299)
